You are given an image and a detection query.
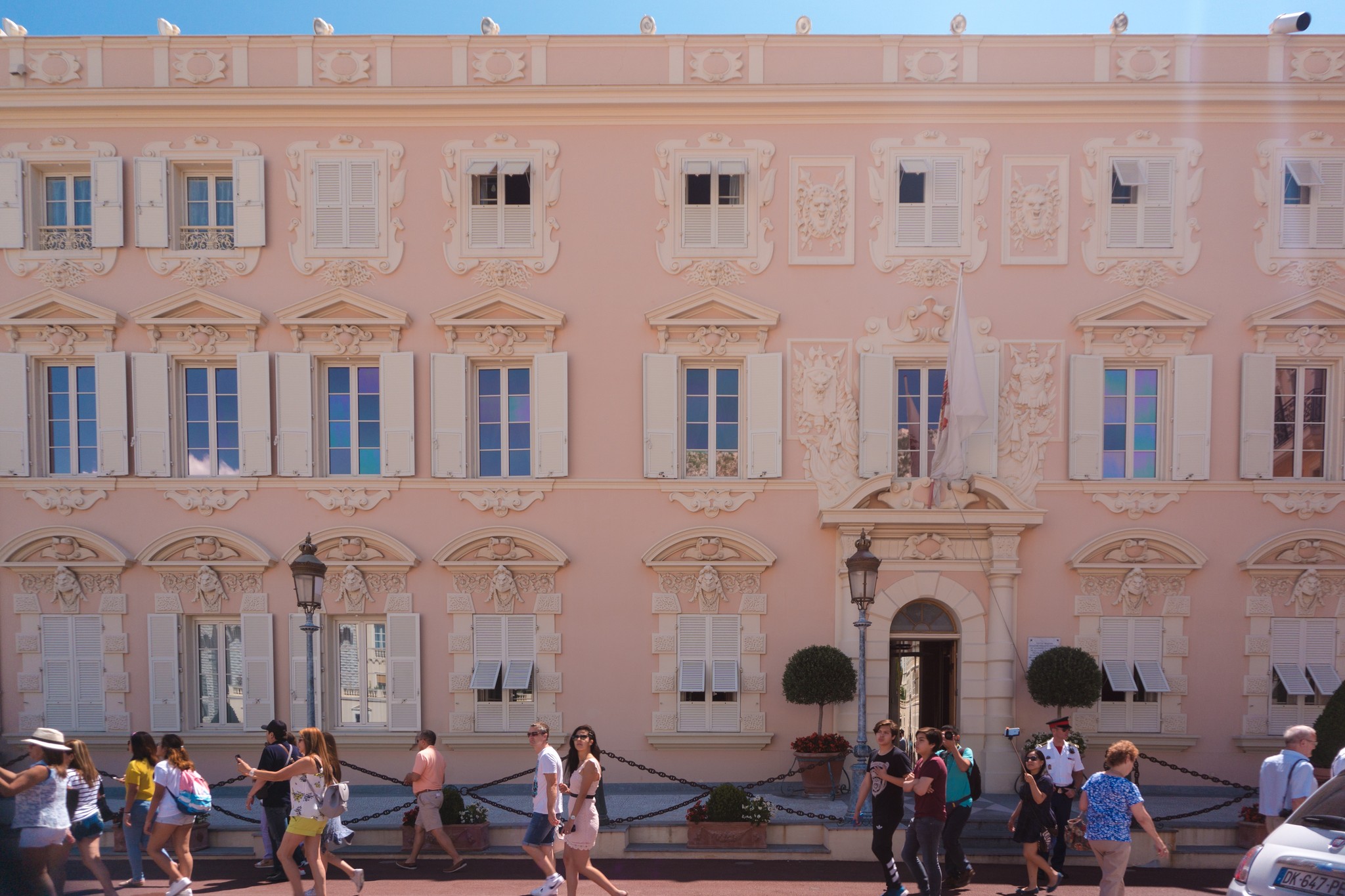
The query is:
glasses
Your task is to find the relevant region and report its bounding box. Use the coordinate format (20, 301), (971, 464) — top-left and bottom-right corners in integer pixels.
(298, 738), (304, 742)
(1300, 738), (1318, 746)
(528, 732), (546, 738)
(572, 735), (592, 742)
(1025, 756), (1038, 762)
(417, 739), (425, 743)
(1055, 728), (1066, 732)
(127, 741), (132, 746)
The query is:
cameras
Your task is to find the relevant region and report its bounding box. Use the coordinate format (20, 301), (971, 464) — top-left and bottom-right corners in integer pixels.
(945, 731), (953, 740)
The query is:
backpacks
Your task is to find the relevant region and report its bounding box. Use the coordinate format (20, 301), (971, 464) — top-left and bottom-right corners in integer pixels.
(166, 767), (212, 815)
(303, 774), (349, 818)
(940, 748), (981, 801)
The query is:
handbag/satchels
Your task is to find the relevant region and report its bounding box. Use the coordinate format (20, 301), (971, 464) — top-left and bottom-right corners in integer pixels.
(945, 796), (970, 816)
(66, 788), (79, 820)
(1038, 827), (1051, 853)
(97, 782), (114, 822)
(1065, 810), (1092, 851)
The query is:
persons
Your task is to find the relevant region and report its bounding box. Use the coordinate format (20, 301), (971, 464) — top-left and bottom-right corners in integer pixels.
(0, 719), (629, 896)
(899, 729), (906, 751)
(853, 716), (1170, 896)
(1258, 725), (1345, 838)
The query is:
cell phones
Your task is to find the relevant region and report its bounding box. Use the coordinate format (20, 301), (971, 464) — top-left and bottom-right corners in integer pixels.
(1004, 728), (1020, 736)
(236, 754), (241, 764)
(852, 813), (864, 828)
(559, 825), (575, 834)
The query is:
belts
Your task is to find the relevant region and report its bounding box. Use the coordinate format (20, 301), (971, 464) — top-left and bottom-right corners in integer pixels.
(1055, 788), (1065, 793)
(415, 789), (441, 798)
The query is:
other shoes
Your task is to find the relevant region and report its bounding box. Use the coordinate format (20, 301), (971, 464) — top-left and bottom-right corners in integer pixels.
(396, 858), (416, 869)
(443, 859), (467, 873)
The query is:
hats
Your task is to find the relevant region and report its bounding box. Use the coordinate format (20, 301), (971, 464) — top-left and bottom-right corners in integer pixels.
(20, 728), (72, 750)
(261, 720), (287, 736)
(1047, 716), (1072, 729)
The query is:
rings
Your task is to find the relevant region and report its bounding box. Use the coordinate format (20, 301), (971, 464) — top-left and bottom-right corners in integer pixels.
(1164, 851), (1167, 853)
(246, 805), (248, 806)
(566, 830), (569, 833)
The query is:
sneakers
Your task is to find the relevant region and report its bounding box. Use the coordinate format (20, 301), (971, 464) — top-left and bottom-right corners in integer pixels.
(882, 866), (1063, 896)
(122, 857), (365, 896)
(531, 873), (565, 896)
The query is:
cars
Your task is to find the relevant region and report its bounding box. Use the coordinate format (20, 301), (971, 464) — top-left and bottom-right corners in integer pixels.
(1222, 765), (1345, 896)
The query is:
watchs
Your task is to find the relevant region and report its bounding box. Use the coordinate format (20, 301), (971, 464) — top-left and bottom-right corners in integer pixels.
(248, 768), (257, 776)
(570, 815), (576, 820)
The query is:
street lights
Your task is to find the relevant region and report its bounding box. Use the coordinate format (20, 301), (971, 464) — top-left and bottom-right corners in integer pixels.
(288, 531), (329, 727)
(843, 526), (883, 822)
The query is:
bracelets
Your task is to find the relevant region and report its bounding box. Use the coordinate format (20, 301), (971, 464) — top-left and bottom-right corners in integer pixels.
(123, 812), (130, 814)
(1072, 787), (1078, 793)
(247, 793), (253, 798)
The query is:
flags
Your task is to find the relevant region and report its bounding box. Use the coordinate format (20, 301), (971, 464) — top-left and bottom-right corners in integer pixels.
(925, 264), (989, 504)
(903, 376), (934, 480)
